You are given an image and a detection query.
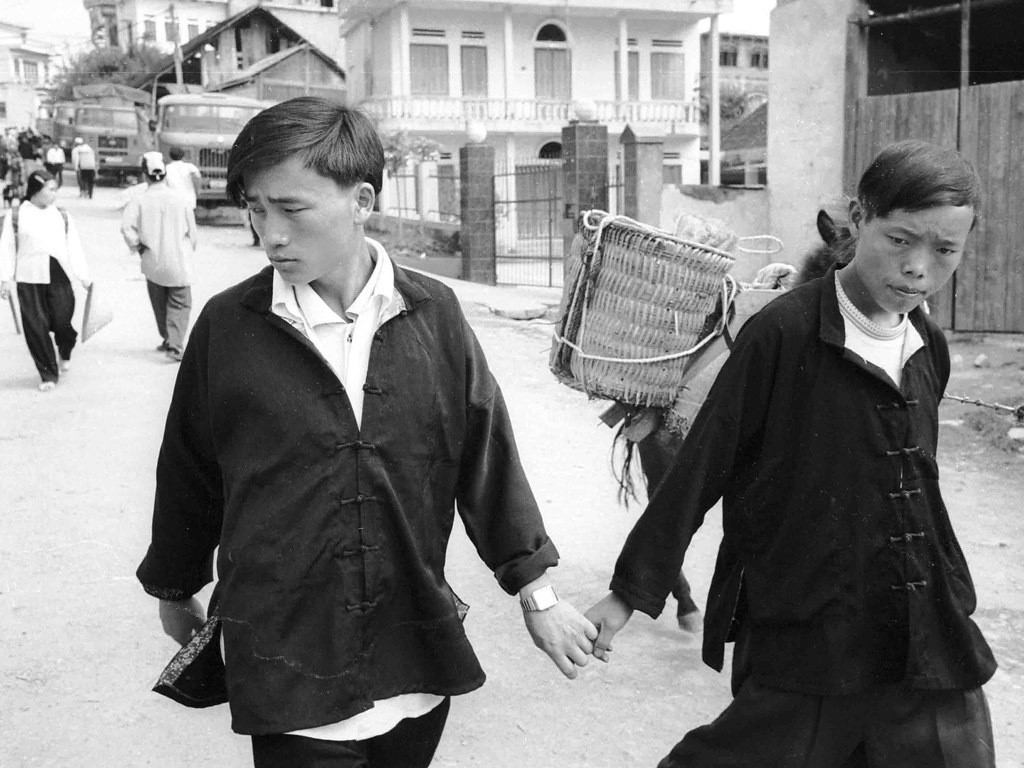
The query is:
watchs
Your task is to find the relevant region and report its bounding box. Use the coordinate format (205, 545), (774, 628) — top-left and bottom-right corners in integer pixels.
(518, 585), (559, 612)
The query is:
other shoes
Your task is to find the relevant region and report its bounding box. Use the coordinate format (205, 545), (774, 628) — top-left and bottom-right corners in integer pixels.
(157, 341), (169, 351)
(39, 381), (55, 391)
(60, 359), (69, 372)
(167, 348), (182, 360)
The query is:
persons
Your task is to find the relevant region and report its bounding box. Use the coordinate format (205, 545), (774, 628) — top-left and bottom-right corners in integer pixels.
(164, 150), (202, 251)
(120, 152), (198, 361)
(138, 96), (598, 768)
(41, 136), (65, 188)
(73, 141), (96, 199)
(585, 142), (998, 768)
(0, 134), (42, 198)
(0, 171), (90, 391)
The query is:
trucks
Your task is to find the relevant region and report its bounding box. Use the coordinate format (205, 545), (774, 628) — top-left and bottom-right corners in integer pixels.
(148, 92), (276, 211)
(29, 84), (158, 188)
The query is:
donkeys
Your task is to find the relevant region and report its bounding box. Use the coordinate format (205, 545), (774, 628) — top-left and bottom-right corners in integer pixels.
(611, 210), (942, 633)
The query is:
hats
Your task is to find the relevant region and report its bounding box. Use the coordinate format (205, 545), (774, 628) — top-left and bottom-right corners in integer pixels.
(81, 282), (113, 342)
(142, 152), (166, 180)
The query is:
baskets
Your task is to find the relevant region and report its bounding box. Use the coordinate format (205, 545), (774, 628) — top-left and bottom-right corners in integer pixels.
(548, 209), (737, 409)
